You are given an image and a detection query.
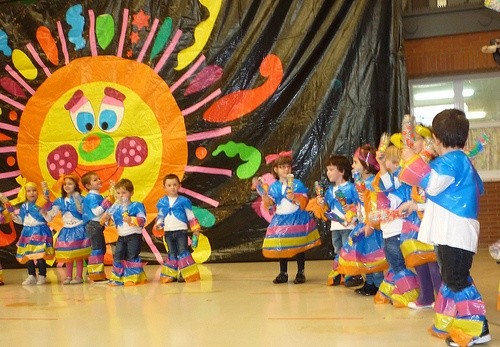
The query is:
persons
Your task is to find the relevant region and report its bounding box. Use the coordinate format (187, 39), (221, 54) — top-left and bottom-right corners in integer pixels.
(0, 212), (13, 285)
(152, 174), (200, 282)
(305, 156), (364, 287)
(100, 179), (147, 286)
(336, 124), (442, 309)
(255, 151), (322, 285)
(81, 172), (116, 284)
(399, 109), (491, 347)
(43, 176), (92, 284)
(6, 175), (54, 285)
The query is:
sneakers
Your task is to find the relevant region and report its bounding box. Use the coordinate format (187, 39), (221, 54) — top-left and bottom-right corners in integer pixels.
(408, 297), (436, 309)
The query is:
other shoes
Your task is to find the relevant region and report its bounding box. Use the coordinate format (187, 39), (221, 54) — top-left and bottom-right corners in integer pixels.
(354, 284), (378, 295)
(294, 269), (306, 284)
(177, 275), (185, 282)
(70, 276), (84, 283)
(273, 273), (288, 283)
(36, 275), (46, 286)
(21, 275), (38, 285)
(446, 324), (491, 347)
(346, 275), (364, 287)
(63, 276), (72, 284)
(332, 274), (341, 284)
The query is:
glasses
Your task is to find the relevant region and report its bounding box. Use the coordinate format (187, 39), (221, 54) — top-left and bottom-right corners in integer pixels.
(276, 165), (292, 170)
